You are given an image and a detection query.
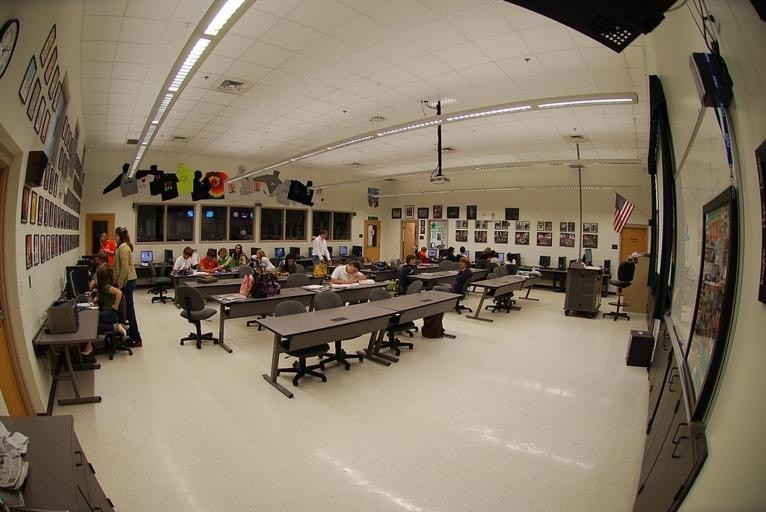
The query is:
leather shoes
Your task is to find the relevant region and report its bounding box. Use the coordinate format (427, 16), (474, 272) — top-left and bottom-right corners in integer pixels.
(81, 346), (94, 356)
(119, 323), (127, 339)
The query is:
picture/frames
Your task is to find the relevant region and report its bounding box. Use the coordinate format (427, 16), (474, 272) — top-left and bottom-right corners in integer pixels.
(19, 24), (87, 269)
(682, 183), (740, 425)
(391, 205), (598, 248)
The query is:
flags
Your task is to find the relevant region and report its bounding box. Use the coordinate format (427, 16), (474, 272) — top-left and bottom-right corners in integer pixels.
(612, 193), (635, 235)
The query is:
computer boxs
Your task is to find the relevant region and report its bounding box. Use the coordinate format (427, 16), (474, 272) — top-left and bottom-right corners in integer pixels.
(439, 249), (449, 257)
(475, 251), (483, 262)
(165, 249), (173, 264)
(510, 253), (520, 266)
(66, 266), (90, 295)
(82, 254), (97, 261)
(290, 247), (300, 257)
(353, 246), (362, 258)
(77, 259), (96, 270)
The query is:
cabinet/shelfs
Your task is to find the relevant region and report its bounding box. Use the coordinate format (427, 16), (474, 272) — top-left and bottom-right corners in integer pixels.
(622, 255), (650, 313)
(563, 267), (602, 318)
(632, 322), (708, 512)
(1, 415), (117, 512)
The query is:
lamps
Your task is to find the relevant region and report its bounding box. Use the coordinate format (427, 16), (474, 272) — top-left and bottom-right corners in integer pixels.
(430, 100), (450, 185)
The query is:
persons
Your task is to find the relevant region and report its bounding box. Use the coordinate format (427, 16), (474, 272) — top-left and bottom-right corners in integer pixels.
(312, 230), (332, 271)
(331, 261), (367, 284)
(111, 226), (142, 348)
(165, 244), (297, 279)
(398, 246), (518, 297)
(80, 232), (129, 357)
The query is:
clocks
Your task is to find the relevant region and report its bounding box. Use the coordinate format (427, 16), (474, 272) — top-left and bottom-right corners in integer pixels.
(0, 19), (20, 79)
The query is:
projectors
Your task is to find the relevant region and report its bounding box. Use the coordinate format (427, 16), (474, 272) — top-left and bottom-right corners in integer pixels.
(431, 176), (451, 186)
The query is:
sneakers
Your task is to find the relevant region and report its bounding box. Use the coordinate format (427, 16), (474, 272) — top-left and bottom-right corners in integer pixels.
(121, 338), (142, 347)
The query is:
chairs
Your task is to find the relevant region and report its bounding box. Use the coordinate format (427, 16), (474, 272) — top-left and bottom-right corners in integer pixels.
(603, 262), (635, 321)
(99, 295), (133, 360)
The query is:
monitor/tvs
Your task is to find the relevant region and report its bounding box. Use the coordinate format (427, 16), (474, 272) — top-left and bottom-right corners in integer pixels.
(207, 248), (216, 260)
(498, 252), (504, 262)
(339, 245), (347, 255)
(66, 271), (79, 299)
(206, 211), (214, 218)
(187, 211), (194, 217)
(428, 249), (437, 257)
(459, 251), (469, 258)
(274, 247), (285, 258)
(141, 251), (154, 262)
(541, 255), (552, 270)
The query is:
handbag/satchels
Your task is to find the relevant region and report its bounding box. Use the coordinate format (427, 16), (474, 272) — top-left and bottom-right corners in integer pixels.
(385, 281), (397, 292)
(314, 263), (328, 278)
(196, 274), (218, 283)
(178, 267), (193, 275)
(371, 261), (392, 271)
(240, 274), (280, 299)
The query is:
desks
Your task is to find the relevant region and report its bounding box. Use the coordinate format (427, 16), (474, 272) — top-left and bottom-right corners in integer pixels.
(518, 265), (612, 297)
(515, 271), (544, 301)
(257, 302), (390, 398)
(32, 299), (102, 406)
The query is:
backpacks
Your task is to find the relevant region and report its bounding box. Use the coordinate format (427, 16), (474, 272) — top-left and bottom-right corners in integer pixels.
(422, 313), (446, 339)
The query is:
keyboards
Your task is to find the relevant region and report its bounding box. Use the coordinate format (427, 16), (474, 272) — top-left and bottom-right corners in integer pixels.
(141, 263), (148, 266)
